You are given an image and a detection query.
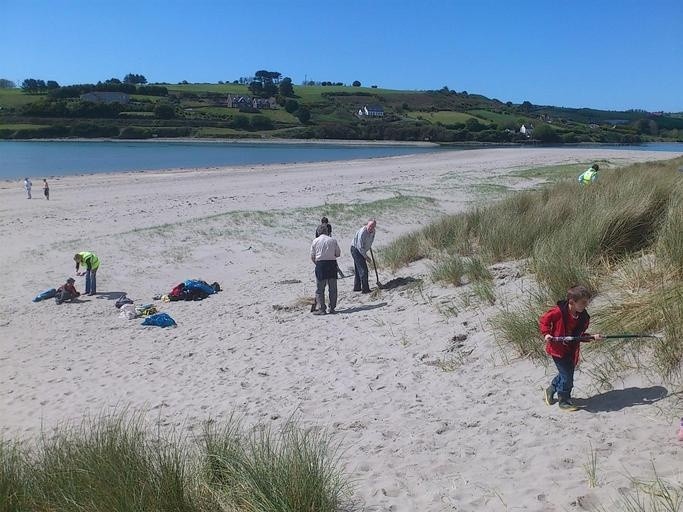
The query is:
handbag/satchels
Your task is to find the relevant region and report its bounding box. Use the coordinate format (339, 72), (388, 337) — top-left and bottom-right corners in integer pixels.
(116, 295), (133, 308)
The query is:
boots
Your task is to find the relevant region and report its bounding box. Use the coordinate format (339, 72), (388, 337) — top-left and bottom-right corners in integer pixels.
(558, 393), (578, 412)
(545, 387), (555, 406)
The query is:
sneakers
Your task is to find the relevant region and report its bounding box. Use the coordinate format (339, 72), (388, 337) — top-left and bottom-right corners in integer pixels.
(327, 309), (337, 314)
(313, 309), (326, 315)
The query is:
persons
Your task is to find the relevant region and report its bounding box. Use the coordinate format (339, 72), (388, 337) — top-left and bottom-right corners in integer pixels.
(349, 218), (376, 294)
(309, 223), (340, 315)
(315, 218), (338, 280)
(23, 178), (32, 198)
(538, 284), (603, 411)
(54, 278), (79, 304)
(72, 252), (100, 296)
(578, 163), (599, 188)
(41, 179), (49, 200)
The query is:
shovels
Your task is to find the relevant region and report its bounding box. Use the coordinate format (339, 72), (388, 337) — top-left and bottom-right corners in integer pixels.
(311, 296), (318, 312)
(370, 248), (384, 289)
